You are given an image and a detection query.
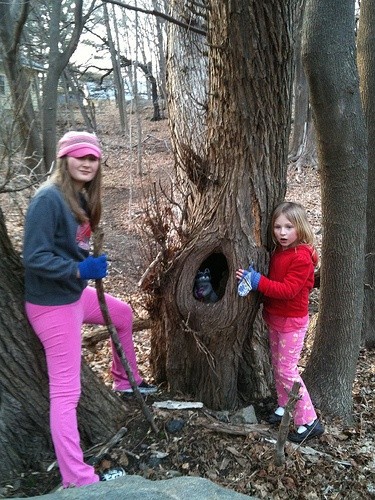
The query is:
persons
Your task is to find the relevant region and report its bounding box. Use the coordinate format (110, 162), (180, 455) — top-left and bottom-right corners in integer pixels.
(21, 131), (158, 490)
(236, 202), (325, 441)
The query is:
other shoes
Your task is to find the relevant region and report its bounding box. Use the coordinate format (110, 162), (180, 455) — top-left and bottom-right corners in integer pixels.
(113, 379), (157, 393)
(96, 465), (126, 482)
(288, 418), (323, 442)
(267, 406), (292, 424)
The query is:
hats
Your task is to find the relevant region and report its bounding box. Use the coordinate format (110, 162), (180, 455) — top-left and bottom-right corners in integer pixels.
(57, 130), (102, 159)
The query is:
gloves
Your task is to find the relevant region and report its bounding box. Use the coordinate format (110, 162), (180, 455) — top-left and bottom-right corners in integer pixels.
(77, 251), (108, 280)
